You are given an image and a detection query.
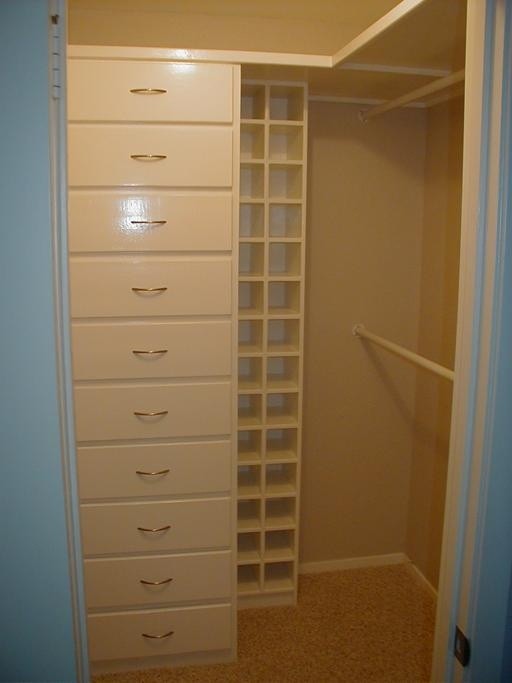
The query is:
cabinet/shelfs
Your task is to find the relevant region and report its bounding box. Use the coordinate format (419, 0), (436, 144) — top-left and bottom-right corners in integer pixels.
(67, 59), (307, 676)
